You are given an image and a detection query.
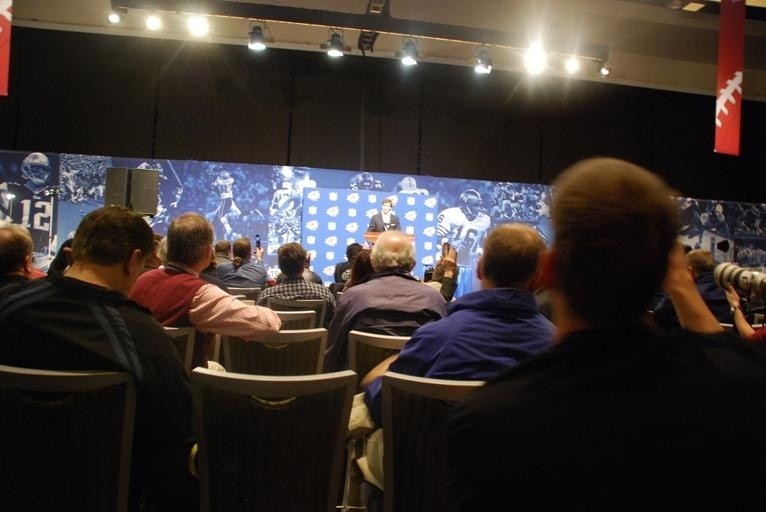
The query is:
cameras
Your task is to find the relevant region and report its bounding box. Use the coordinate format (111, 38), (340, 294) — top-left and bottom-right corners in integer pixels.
(440, 242), (450, 257)
(713, 259), (766, 304)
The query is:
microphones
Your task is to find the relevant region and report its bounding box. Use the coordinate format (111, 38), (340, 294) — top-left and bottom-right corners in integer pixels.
(384, 223), (387, 230)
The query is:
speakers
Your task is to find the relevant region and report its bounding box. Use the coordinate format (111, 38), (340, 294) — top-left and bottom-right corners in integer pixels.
(127, 168), (160, 216)
(103, 166), (127, 210)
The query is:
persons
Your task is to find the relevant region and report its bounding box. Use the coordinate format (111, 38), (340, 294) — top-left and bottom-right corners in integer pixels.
(397, 175), (430, 197)
(487, 180), (550, 225)
(358, 223), (559, 487)
(0, 202), (201, 511)
(438, 189), (491, 257)
(723, 282), (766, 344)
(349, 173), (384, 191)
(655, 248), (733, 324)
(213, 170), (234, 236)
(675, 196), (766, 267)
(0, 153), (60, 273)
(61, 164), (80, 205)
(125, 210), (282, 340)
(367, 199), (401, 233)
(319, 230), (460, 372)
(1, 221), (37, 285)
(448, 155), (765, 510)
(254, 242), (337, 329)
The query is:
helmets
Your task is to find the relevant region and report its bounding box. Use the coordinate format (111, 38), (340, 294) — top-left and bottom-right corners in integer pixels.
(359, 172), (373, 189)
(458, 189), (487, 219)
(399, 174), (418, 190)
(22, 152), (50, 185)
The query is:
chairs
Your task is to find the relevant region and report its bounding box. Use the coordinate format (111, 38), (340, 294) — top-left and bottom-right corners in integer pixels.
(222, 327), (329, 372)
(238, 300), (256, 306)
(1, 365), (137, 511)
(192, 364), (358, 512)
(268, 295), (328, 330)
(341, 331), (414, 391)
(213, 308), (317, 363)
(335, 290), (345, 302)
(381, 372), (486, 512)
(162, 324), (194, 378)
(226, 286), (263, 296)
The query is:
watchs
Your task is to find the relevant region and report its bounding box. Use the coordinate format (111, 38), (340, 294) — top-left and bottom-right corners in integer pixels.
(729, 306), (742, 315)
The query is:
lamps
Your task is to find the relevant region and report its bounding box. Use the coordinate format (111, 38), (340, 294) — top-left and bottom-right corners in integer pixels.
(474, 47), (493, 76)
(599, 51), (613, 76)
(108, 7), (126, 24)
(400, 38), (419, 67)
(248, 24), (268, 52)
(328, 30), (345, 57)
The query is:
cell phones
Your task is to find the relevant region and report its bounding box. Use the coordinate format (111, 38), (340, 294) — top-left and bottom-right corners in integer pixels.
(255, 234), (260, 250)
